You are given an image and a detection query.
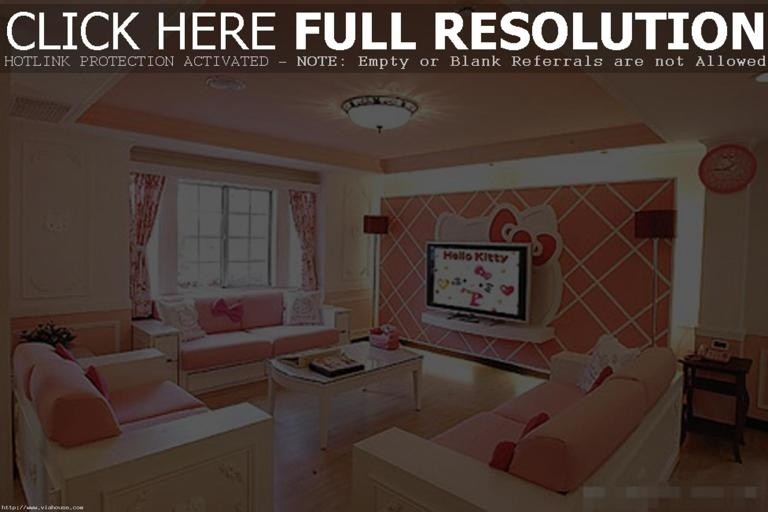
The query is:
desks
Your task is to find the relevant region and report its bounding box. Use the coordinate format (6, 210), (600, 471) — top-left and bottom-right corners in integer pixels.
(679, 352), (752, 462)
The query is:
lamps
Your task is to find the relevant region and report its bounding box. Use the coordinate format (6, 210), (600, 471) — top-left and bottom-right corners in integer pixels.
(634, 208), (677, 347)
(363, 213), (389, 332)
(340, 94), (420, 132)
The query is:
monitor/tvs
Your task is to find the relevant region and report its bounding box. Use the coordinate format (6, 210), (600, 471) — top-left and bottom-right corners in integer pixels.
(425, 243), (532, 325)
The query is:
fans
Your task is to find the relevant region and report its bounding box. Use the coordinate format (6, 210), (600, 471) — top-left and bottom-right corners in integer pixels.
(265, 340), (423, 448)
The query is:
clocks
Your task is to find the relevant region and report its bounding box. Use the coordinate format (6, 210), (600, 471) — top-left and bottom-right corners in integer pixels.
(697, 143), (756, 195)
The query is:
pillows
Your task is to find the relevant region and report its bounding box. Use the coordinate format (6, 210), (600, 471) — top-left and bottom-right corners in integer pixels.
(576, 334), (633, 395)
(281, 291), (325, 326)
(156, 297), (210, 341)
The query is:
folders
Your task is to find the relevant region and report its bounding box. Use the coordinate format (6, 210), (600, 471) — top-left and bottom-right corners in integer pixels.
(276, 348), (341, 369)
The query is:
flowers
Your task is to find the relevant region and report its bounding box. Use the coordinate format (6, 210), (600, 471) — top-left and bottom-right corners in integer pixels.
(18, 317), (76, 350)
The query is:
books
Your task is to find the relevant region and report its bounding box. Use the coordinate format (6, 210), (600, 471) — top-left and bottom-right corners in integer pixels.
(308, 353), (365, 377)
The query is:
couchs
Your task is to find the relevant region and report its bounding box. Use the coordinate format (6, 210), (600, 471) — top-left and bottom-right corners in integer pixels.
(130, 288), (351, 400)
(12, 340), (274, 511)
(352, 348), (686, 510)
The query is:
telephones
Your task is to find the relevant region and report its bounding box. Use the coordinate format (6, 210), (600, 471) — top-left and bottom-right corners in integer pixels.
(696, 339), (731, 363)
